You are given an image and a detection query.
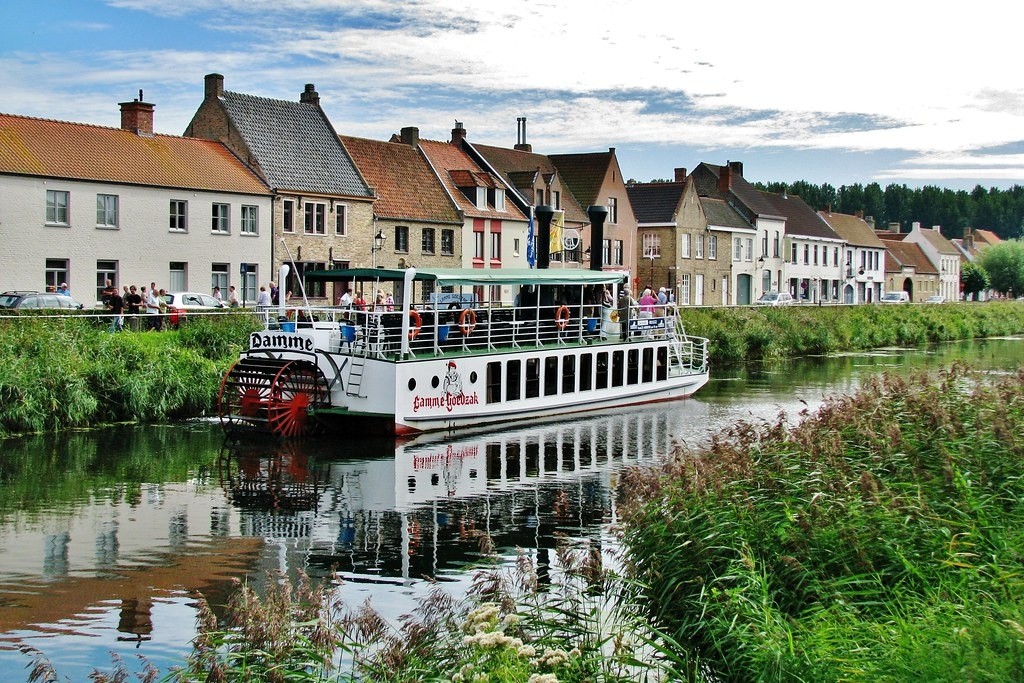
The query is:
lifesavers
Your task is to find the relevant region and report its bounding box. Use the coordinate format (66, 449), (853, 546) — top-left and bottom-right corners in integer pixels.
(406, 311), (423, 339)
(286, 310), (304, 322)
(458, 309), (477, 335)
(556, 306), (571, 329)
(347, 302), (359, 311)
(448, 303), (461, 310)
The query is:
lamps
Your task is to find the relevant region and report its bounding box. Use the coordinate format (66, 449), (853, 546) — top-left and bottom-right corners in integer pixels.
(755, 256), (765, 268)
(585, 246), (592, 259)
(859, 266), (865, 275)
(372, 229), (387, 253)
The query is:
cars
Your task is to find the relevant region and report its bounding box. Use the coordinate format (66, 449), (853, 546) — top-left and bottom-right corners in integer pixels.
(0, 291), (84, 311)
(924, 295), (948, 304)
(880, 290), (909, 305)
(162, 291), (230, 309)
(755, 291), (796, 306)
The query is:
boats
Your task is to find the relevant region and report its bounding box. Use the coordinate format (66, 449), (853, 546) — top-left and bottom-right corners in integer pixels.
(217, 257), (711, 440)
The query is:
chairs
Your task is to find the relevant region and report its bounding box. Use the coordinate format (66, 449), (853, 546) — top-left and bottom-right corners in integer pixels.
(277, 316), (288, 328)
(305, 315), (319, 328)
(338, 319), (365, 354)
(295, 315), (308, 328)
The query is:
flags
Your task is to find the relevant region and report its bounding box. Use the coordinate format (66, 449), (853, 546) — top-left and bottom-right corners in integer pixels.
(526, 211), (564, 270)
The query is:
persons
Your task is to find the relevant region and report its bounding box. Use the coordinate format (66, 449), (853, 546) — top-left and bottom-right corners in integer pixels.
(515, 284), (674, 342)
(50, 280), (394, 334)
